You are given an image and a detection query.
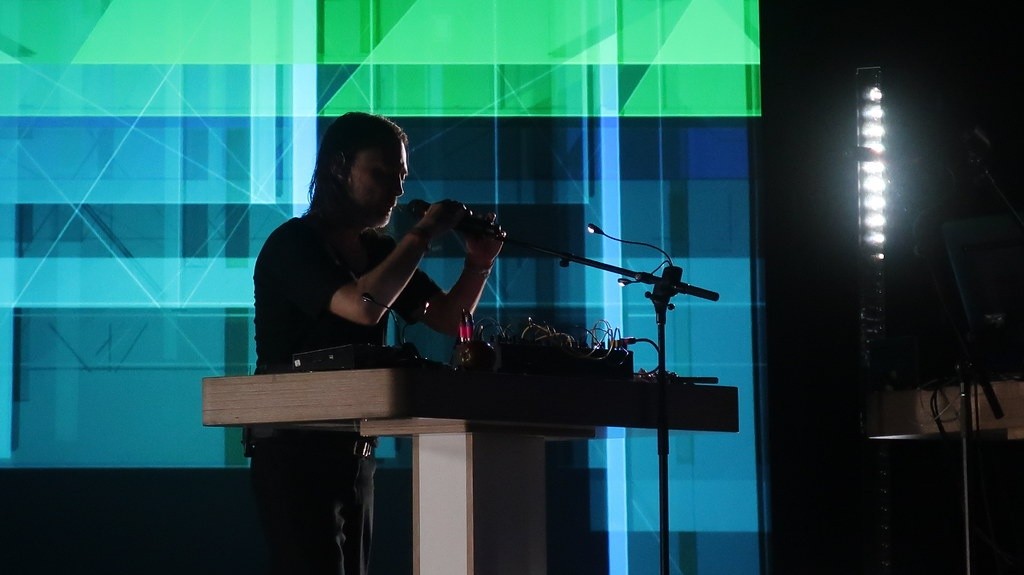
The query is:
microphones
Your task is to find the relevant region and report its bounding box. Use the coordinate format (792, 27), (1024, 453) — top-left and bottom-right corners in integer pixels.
(406, 198), (494, 230)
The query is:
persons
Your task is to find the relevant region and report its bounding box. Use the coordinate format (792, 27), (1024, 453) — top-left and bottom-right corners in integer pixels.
(252, 112), (508, 575)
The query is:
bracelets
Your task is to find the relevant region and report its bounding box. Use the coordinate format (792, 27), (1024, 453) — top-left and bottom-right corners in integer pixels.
(409, 226), (432, 242)
(464, 257), (494, 278)
